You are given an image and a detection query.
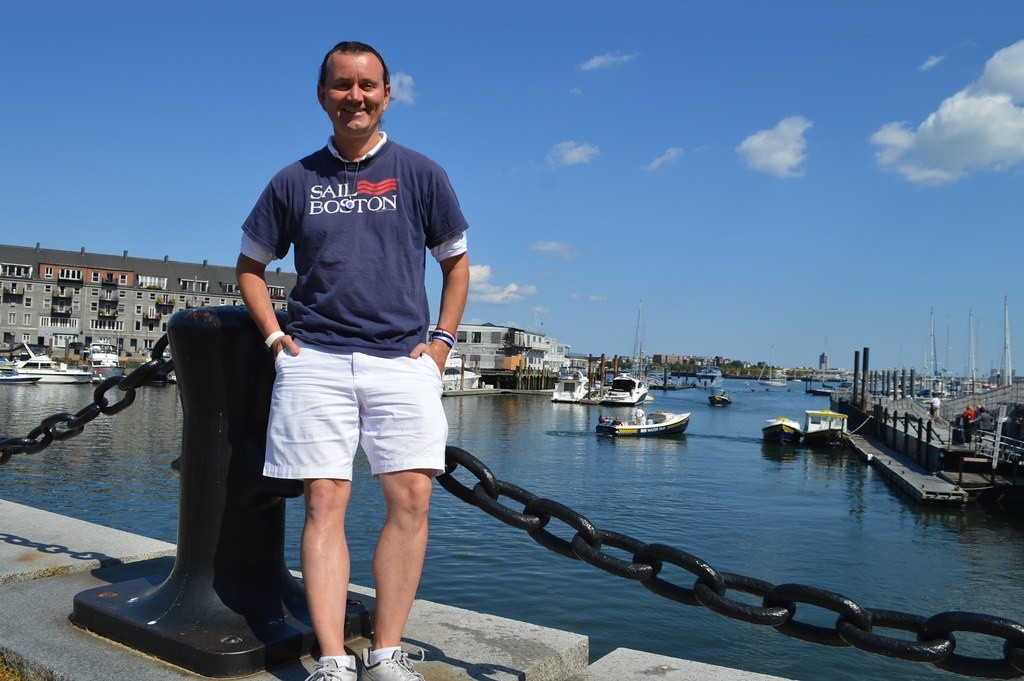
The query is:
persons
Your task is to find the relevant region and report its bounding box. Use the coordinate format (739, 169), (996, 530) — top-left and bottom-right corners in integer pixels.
(963, 405), (995, 443)
(930, 394), (940, 417)
(635, 406), (644, 425)
(599, 415), (624, 426)
(236, 40), (471, 681)
(647, 417), (653, 425)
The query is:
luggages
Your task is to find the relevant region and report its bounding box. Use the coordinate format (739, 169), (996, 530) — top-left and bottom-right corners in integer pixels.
(952, 428), (964, 447)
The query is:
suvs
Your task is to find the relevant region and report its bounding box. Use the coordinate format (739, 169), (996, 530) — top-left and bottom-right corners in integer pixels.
(993, 402), (1024, 465)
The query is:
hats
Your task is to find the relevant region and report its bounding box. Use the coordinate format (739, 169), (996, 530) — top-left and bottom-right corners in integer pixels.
(967, 405), (973, 408)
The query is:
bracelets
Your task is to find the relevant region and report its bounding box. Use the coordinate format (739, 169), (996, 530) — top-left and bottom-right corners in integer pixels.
(432, 328), (455, 349)
(265, 331), (285, 348)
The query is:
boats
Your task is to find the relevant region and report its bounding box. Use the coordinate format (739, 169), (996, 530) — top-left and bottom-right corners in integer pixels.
(839, 379), (853, 388)
(867, 305), (1003, 405)
(604, 297), (726, 391)
(708, 390), (732, 407)
(600, 372), (648, 407)
(761, 416), (804, 446)
(595, 408), (692, 437)
(0, 328), (169, 388)
(441, 340), (482, 395)
(805, 369), (835, 396)
(550, 368), (595, 403)
(802, 409), (850, 449)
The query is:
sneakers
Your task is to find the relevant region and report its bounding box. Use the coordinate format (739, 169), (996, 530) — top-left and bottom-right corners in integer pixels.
(361, 646), (424, 681)
(305, 655), (358, 681)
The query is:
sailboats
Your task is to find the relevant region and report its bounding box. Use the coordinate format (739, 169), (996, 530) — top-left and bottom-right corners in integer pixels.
(757, 344), (787, 387)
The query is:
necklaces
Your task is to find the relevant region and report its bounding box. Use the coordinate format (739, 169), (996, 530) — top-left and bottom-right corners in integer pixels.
(343, 155), (359, 199)
